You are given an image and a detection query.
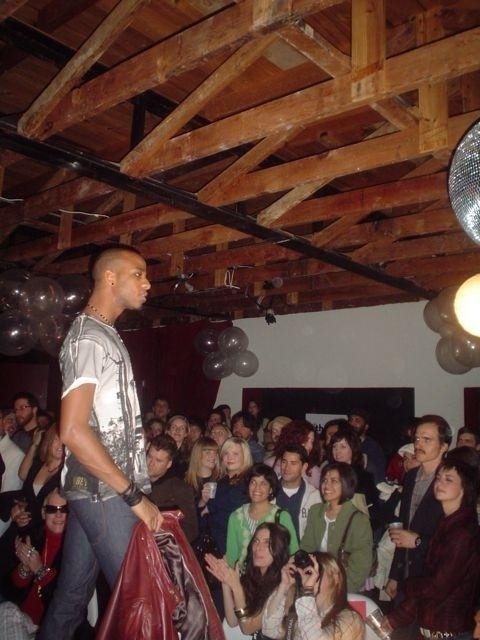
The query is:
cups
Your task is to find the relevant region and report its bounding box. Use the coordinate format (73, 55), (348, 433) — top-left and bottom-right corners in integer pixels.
(363, 609), (393, 639)
(205, 482), (218, 500)
(388, 522), (404, 543)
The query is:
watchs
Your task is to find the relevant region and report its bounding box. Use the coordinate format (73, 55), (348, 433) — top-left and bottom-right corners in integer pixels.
(415, 535), (423, 548)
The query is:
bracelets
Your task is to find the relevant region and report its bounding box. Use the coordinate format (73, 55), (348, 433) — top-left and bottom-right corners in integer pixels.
(300, 586), (314, 596)
(199, 504), (207, 513)
(36, 566), (49, 581)
(18, 562), (34, 577)
(234, 606), (250, 620)
(118, 479), (144, 507)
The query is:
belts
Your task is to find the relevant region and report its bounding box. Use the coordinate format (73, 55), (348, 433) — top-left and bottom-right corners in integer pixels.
(418, 627), (465, 640)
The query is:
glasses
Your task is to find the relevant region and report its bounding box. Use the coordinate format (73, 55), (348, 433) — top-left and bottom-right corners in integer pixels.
(42, 504), (69, 514)
(167, 424), (188, 432)
(209, 430), (226, 437)
(12, 405), (33, 412)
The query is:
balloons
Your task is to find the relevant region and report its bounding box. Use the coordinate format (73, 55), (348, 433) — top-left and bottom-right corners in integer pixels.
(228, 350), (258, 377)
(193, 328), (220, 357)
(0, 268), (93, 357)
(202, 351), (233, 379)
(217, 326), (248, 354)
(423, 283), (480, 375)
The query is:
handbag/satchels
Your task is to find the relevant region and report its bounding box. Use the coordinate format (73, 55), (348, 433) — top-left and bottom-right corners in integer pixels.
(375, 481), (403, 518)
(366, 485), (402, 525)
(338, 547), (377, 577)
(193, 535), (222, 590)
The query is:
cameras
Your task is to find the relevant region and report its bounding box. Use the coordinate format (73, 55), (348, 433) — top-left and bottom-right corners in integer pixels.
(289, 549), (314, 587)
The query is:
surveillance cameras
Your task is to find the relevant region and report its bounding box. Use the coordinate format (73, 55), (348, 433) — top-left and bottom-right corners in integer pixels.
(265, 308), (276, 326)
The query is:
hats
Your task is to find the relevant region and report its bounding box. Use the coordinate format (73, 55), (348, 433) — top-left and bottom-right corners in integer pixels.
(348, 406), (370, 422)
(268, 416), (293, 431)
(397, 443), (415, 458)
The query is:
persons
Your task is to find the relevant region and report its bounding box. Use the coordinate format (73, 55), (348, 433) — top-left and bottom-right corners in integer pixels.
(34, 410), (53, 438)
(185, 415), (206, 451)
(165, 415), (191, 483)
(269, 443), (323, 544)
(196, 435), (252, 558)
(203, 522), (295, 640)
(348, 407), (385, 483)
(203, 409), (227, 434)
(142, 408), (156, 427)
(300, 462), (374, 595)
(145, 417), (164, 441)
(371, 414), (454, 616)
(36, 243), (163, 640)
(1, 405), (39, 441)
(326, 428), (385, 532)
(312, 433), (329, 467)
(205, 422), (233, 449)
(140, 423), (153, 454)
(319, 419), (349, 446)
(262, 551), (365, 640)
(0, 422), (66, 607)
(386, 459), (479, 640)
(263, 416), (293, 463)
(471, 596), (480, 640)
(183, 435), (219, 504)
(11, 487), (69, 639)
(263, 420), (322, 491)
(216, 404), (232, 430)
(45, 408), (59, 425)
(230, 411), (266, 467)
(385, 417), (422, 485)
(245, 399), (270, 448)
(385, 443), (421, 525)
(9, 391), (47, 490)
(456, 424), (480, 451)
(151, 395), (172, 423)
(223, 463), (300, 563)
(145, 434), (202, 542)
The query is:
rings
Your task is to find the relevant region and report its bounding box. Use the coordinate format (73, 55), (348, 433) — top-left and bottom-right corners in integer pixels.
(32, 546), (36, 552)
(27, 549), (32, 555)
(27, 553), (31, 560)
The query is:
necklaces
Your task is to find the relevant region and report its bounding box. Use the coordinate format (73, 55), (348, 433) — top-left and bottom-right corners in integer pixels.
(85, 304), (115, 329)
